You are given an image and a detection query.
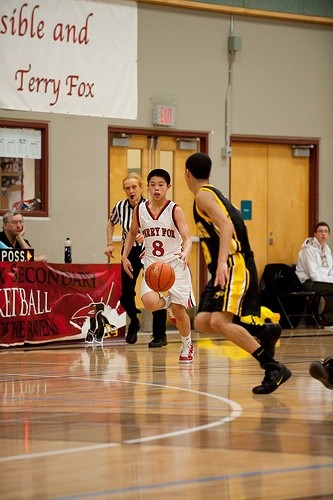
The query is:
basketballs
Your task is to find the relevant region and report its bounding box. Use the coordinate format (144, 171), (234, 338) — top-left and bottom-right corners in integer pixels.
(145, 262), (175, 292)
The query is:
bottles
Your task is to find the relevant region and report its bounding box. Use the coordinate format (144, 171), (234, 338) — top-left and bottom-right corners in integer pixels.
(64, 237), (72, 263)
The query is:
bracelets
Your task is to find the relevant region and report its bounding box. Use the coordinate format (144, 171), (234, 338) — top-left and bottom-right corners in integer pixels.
(106, 244), (114, 247)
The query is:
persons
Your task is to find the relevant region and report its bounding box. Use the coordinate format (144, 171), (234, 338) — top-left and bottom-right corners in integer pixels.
(295, 222), (333, 327)
(122, 168), (197, 363)
(0, 209), (32, 251)
(104, 172), (169, 348)
(185, 153), (292, 394)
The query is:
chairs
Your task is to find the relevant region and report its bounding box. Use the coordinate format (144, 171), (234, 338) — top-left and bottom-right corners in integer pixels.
(262, 263), (322, 329)
